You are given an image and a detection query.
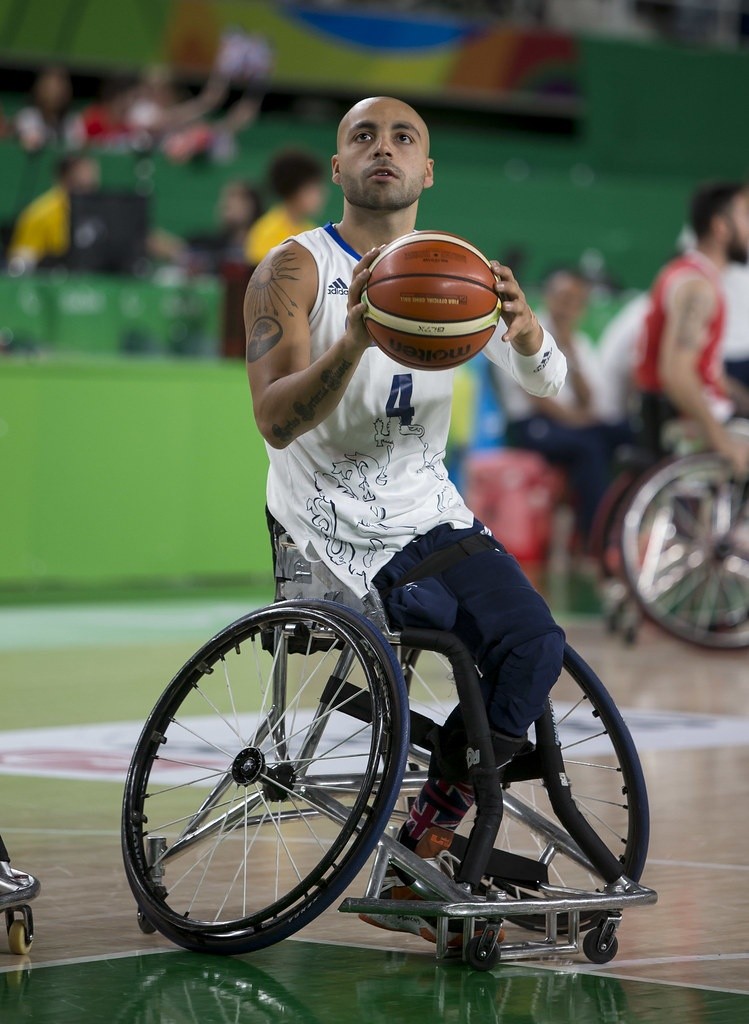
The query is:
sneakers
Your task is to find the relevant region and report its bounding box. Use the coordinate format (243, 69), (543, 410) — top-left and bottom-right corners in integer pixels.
(358, 849), (506, 948)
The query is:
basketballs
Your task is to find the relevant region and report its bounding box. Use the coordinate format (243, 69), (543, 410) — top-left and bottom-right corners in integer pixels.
(358, 229), (503, 372)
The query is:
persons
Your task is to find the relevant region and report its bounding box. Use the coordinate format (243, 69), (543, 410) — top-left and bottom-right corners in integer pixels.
(0, 33), (749, 944)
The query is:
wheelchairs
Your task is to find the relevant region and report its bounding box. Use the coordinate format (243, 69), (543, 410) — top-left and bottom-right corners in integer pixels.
(117, 491), (659, 968)
(603, 409), (749, 653)
